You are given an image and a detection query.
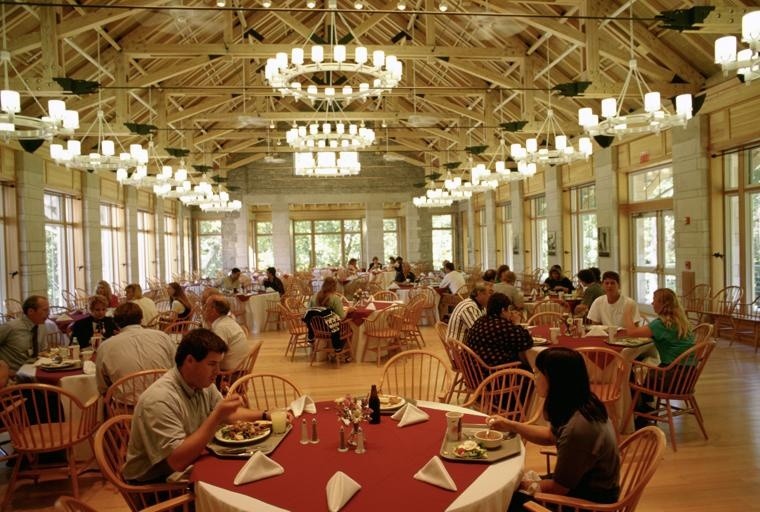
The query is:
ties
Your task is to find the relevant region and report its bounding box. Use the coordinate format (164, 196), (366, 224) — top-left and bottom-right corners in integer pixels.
(30, 324), (38, 358)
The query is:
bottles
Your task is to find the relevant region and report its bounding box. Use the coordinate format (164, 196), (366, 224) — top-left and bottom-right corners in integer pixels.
(367, 384), (382, 426)
(70, 335), (80, 345)
(299, 417), (365, 456)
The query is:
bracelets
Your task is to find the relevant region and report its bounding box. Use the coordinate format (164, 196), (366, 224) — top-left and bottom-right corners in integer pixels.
(261, 409), (269, 420)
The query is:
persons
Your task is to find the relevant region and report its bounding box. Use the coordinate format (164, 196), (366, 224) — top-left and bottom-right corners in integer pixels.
(586, 271), (642, 327)
(0, 281), (193, 430)
(119, 327), (295, 512)
(435, 260), (535, 412)
(484, 346), (621, 511)
(574, 267), (606, 324)
(262, 267), (285, 297)
(189, 286), (250, 395)
(615, 289), (699, 432)
(213, 267), (242, 289)
(306, 276), (357, 363)
(544, 264), (575, 294)
(347, 253), (419, 283)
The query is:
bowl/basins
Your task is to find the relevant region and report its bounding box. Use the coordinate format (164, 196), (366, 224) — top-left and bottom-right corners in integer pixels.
(475, 430), (503, 450)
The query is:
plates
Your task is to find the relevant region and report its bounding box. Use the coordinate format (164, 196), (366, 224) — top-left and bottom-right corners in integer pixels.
(215, 425), (271, 443)
(41, 361), (76, 368)
(376, 393), (406, 411)
(531, 335), (546, 346)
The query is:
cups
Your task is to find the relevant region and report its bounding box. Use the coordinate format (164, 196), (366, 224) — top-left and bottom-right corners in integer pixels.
(67, 337), (102, 360)
(548, 317), (619, 345)
(270, 408), (288, 436)
(445, 411), (463, 441)
(558, 290), (577, 300)
